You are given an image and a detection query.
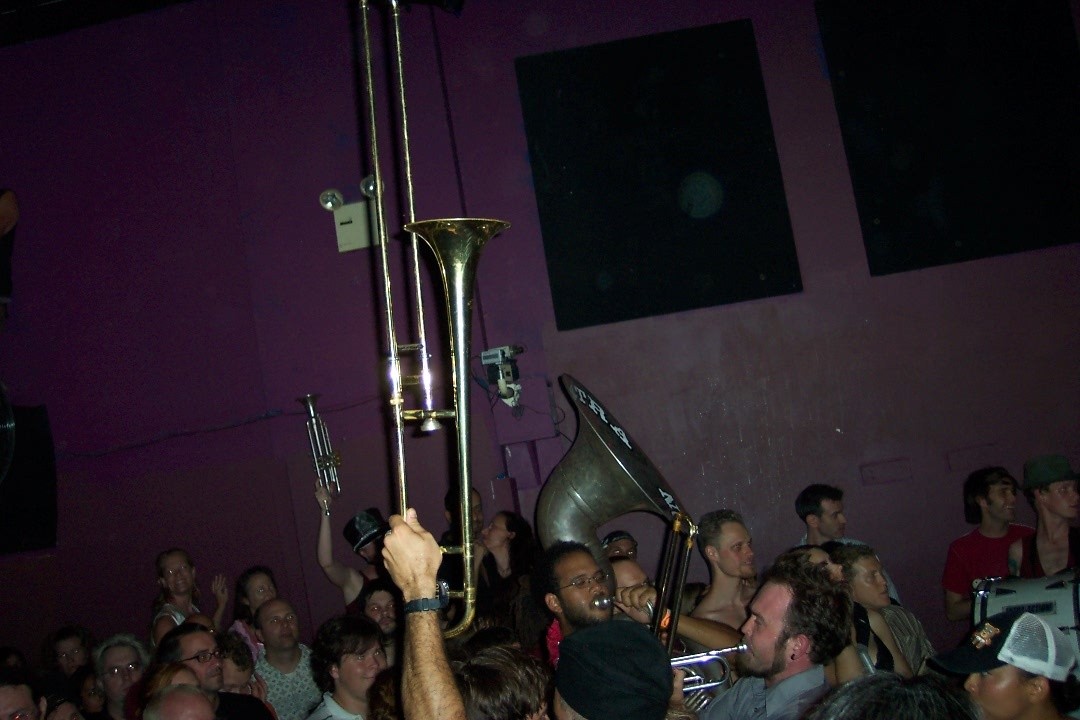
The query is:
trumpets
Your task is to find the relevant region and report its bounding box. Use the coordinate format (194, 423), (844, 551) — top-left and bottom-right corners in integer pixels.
(669, 643), (748, 691)
(295, 391), (342, 517)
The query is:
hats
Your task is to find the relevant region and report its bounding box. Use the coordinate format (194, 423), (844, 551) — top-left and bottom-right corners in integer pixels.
(927, 608), (1075, 682)
(343, 507), (390, 554)
(1022, 457), (1080, 491)
(601, 531), (633, 546)
(554, 619), (673, 719)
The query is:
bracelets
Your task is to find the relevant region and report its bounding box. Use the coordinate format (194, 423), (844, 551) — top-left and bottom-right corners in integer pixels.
(403, 597), (440, 613)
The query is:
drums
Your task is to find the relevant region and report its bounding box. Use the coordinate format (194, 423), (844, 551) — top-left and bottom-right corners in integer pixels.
(973, 572), (1080, 720)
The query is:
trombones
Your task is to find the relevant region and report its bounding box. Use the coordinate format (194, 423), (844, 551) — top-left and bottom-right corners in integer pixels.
(653, 513), (696, 654)
(360, 0), (515, 643)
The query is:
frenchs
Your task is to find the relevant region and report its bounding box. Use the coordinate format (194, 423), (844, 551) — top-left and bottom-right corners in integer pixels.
(532, 370), (714, 714)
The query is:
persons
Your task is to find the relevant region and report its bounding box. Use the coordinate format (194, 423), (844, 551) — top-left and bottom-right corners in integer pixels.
(0, 458), (1080, 720)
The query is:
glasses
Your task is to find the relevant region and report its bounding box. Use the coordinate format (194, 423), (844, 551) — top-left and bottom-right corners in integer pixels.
(102, 662), (139, 676)
(609, 549), (636, 560)
(559, 569), (609, 591)
(59, 648), (82, 660)
(617, 581), (656, 589)
(9, 704), (38, 720)
(177, 648), (225, 664)
